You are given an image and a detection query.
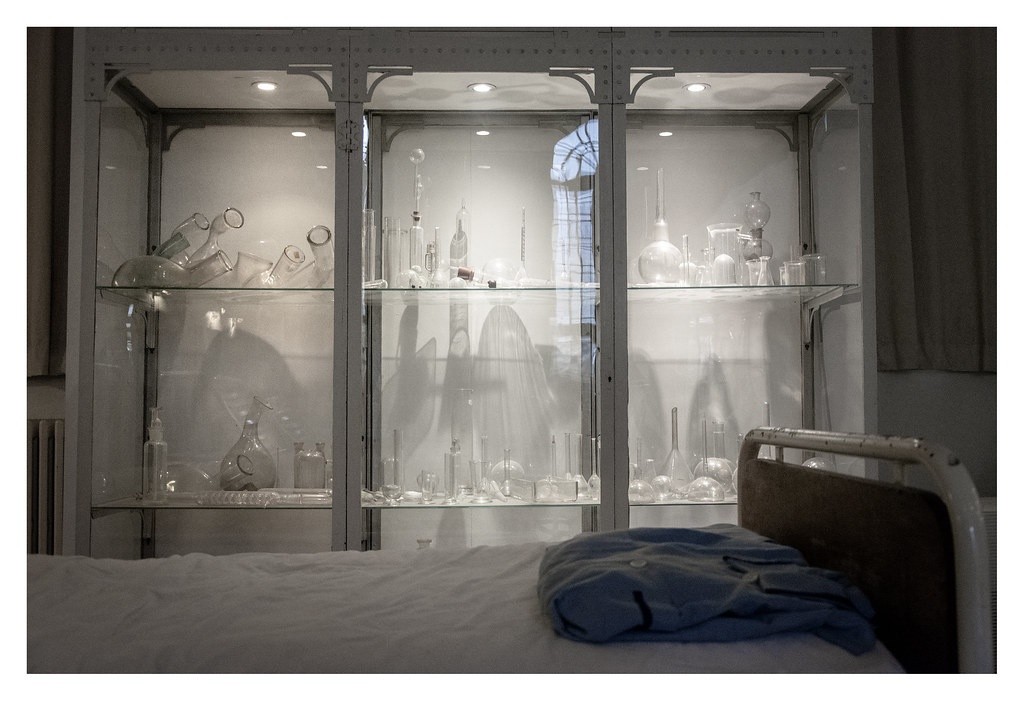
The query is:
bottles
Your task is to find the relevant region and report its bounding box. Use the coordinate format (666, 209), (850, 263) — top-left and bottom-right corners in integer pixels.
(107, 207), (334, 290)
(756, 255), (775, 286)
(219, 394), (277, 494)
(499, 400), (775, 501)
(639, 163), (707, 286)
(446, 436), (461, 502)
(408, 210), (508, 288)
(142, 403), (170, 505)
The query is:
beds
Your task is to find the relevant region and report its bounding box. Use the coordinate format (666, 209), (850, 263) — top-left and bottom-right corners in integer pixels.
(27, 524), (909, 676)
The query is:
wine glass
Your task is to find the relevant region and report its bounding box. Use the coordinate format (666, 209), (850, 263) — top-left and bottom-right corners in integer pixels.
(378, 457), (405, 508)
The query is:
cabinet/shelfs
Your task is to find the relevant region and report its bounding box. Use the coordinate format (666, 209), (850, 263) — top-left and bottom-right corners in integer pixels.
(56, 31), (881, 559)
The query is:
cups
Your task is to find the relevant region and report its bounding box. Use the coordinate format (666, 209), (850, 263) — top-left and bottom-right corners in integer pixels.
(469, 460), (494, 504)
(778, 252), (827, 287)
(362, 207), (407, 290)
(416, 469), (440, 506)
(706, 221), (760, 287)
